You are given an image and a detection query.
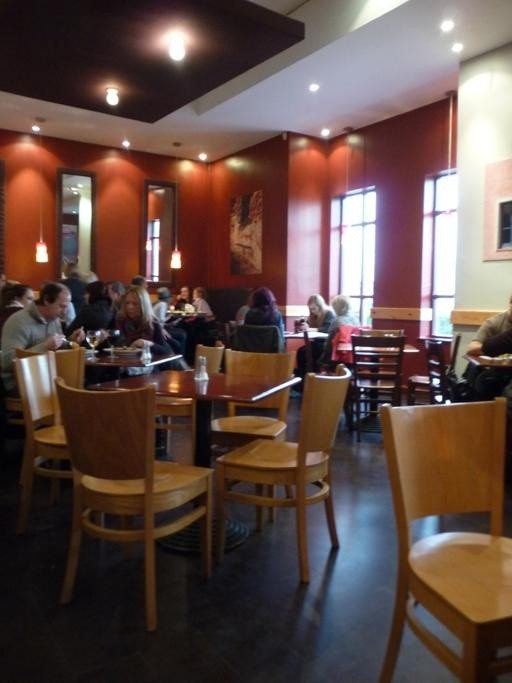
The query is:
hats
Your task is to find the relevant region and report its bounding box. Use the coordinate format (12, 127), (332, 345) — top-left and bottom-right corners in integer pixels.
(157, 287), (171, 299)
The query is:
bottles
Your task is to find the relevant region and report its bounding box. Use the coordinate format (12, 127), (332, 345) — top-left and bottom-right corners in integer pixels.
(139, 341), (152, 364)
(193, 356), (210, 381)
(294, 317), (305, 332)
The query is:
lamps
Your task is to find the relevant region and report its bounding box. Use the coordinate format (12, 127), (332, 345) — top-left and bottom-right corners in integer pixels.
(105, 87), (120, 106)
(32, 116), (51, 263)
(170, 142), (184, 271)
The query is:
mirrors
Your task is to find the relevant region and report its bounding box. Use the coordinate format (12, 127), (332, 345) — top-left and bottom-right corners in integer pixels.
(54, 165), (99, 283)
(140, 176), (180, 290)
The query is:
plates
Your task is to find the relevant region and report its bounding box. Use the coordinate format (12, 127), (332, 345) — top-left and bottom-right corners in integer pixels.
(170, 312), (184, 315)
(185, 312), (195, 315)
(103, 346), (146, 357)
(57, 349), (98, 359)
(197, 312), (208, 315)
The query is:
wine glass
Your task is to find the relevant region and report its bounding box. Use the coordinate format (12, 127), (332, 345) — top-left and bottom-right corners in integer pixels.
(86, 330), (101, 360)
(104, 328), (122, 359)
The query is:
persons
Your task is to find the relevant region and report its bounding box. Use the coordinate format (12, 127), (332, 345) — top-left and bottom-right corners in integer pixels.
(97, 286), (173, 461)
(466, 293), (512, 401)
(134, 276), (215, 350)
(1, 283), (86, 400)
(3, 264), (124, 337)
(481, 310), (511, 401)
(294, 294), (360, 390)
(238, 286), (284, 341)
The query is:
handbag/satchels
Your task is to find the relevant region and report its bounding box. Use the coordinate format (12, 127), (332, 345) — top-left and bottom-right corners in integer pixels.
(447, 374), (474, 402)
